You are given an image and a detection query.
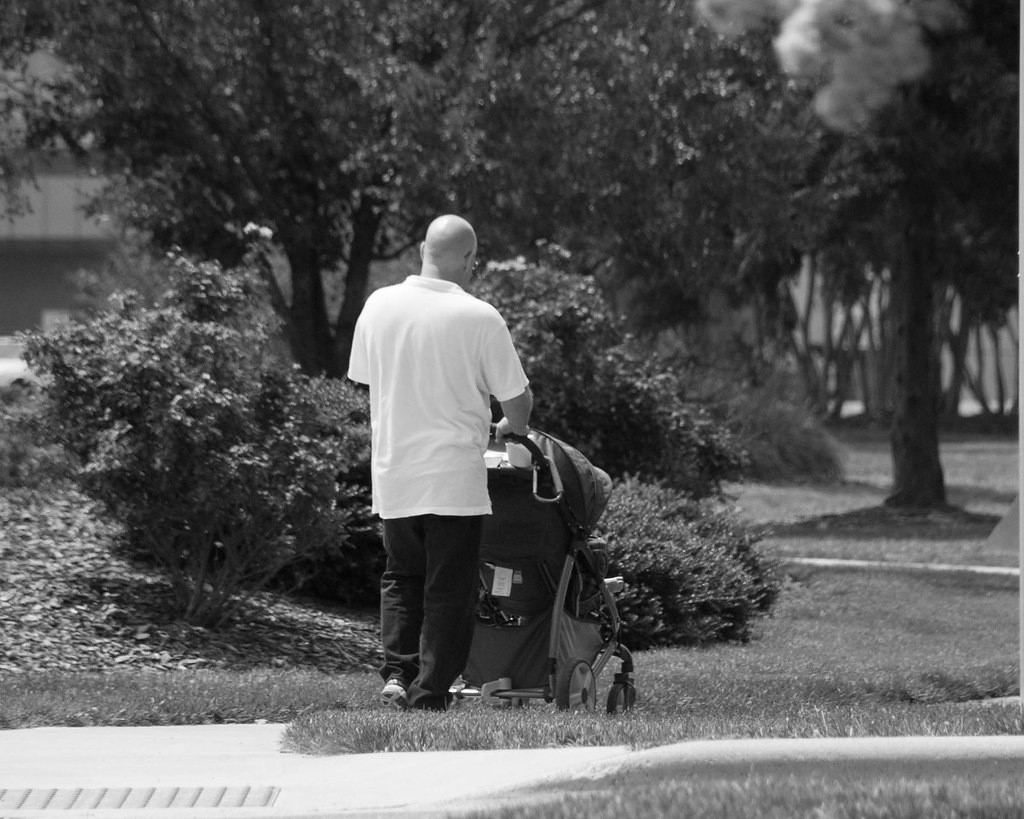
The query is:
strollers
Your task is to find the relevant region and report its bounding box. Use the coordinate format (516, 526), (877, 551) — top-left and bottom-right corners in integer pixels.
(447, 422), (638, 716)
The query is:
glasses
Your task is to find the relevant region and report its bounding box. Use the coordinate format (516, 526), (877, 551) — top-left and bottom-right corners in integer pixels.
(463, 251), (479, 271)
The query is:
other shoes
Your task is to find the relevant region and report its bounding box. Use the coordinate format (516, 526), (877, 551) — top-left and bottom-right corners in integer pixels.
(380, 678), (409, 709)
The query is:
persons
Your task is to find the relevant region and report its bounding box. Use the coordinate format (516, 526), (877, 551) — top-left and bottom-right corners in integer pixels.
(347, 214), (534, 709)
(604, 577), (623, 593)
